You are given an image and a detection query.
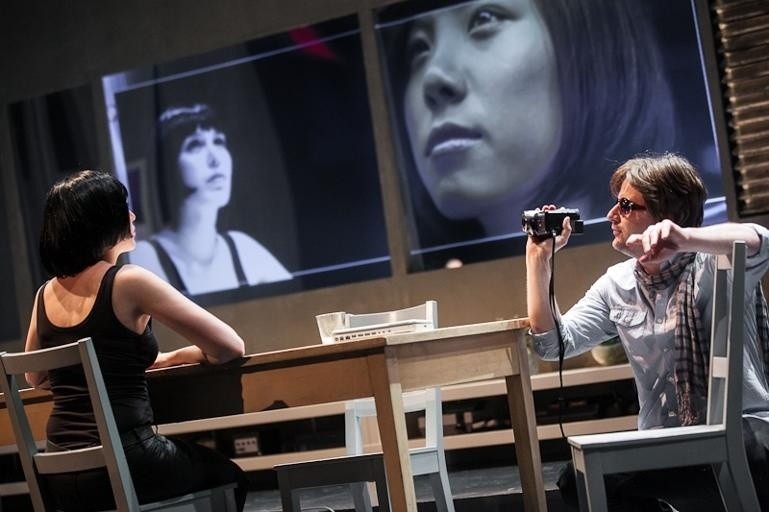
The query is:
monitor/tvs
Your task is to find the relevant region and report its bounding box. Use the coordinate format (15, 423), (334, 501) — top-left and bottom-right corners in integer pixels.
(103, 13), (392, 309)
(370, 0), (728, 274)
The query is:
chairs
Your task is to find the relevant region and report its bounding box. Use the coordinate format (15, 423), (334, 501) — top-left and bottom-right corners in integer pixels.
(272, 300), (455, 512)
(566, 240), (764, 512)
(1, 337), (239, 512)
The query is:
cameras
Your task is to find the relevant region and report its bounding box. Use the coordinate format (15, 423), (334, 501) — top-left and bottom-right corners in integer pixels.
(521, 207), (583, 241)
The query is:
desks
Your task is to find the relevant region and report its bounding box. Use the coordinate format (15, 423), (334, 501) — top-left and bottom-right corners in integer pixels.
(0, 315), (551, 512)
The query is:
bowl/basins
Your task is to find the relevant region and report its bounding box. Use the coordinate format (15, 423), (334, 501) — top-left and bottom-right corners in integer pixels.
(590, 343), (627, 366)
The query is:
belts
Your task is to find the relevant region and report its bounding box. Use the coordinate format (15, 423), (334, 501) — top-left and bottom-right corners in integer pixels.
(113, 424), (156, 446)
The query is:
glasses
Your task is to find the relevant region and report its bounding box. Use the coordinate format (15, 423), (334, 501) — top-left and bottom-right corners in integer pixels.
(610, 194), (644, 219)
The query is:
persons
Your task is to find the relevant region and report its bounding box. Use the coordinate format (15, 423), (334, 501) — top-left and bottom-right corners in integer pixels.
(521, 148), (769, 510)
(396, 0), (712, 259)
(22, 169), (251, 512)
(128, 102), (292, 296)
